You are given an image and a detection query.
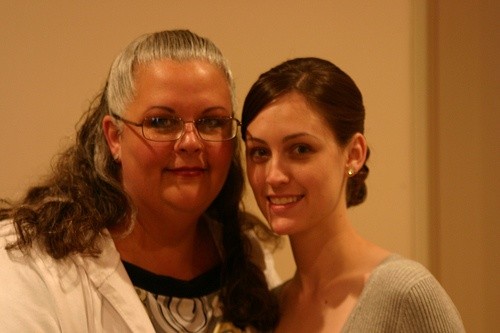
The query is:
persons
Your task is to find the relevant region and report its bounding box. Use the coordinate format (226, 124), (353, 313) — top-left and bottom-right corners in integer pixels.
(240, 57), (465, 333)
(0, 28), (286, 333)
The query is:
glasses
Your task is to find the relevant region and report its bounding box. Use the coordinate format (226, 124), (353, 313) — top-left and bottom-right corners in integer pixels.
(113, 112), (240, 141)
